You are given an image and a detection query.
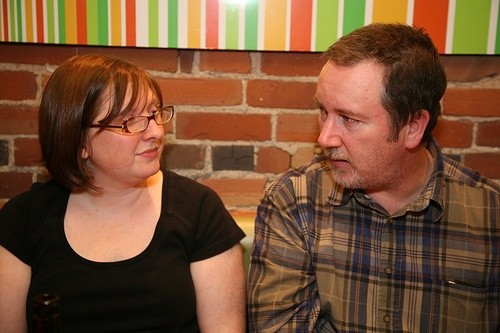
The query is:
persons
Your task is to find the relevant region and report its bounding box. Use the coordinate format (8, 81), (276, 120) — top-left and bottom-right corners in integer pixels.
(0, 55), (247, 333)
(248, 21), (500, 333)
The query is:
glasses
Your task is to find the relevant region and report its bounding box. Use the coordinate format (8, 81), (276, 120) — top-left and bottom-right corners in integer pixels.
(85, 105), (174, 133)
